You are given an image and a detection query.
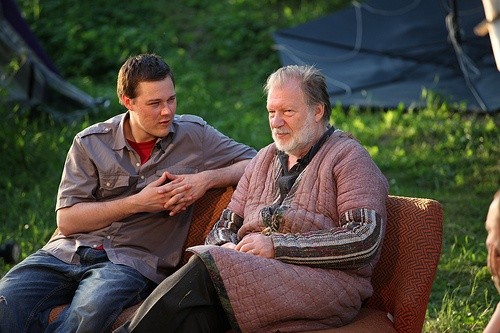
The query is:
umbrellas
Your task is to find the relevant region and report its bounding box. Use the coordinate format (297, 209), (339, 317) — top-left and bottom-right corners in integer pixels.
(271, 0), (500, 112)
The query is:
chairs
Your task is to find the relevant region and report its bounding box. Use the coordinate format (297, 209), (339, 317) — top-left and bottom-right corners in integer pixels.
(49, 186), (443, 333)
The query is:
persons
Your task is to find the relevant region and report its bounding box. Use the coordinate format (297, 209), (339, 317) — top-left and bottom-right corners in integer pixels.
(112, 66), (389, 333)
(0, 54), (258, 333)
